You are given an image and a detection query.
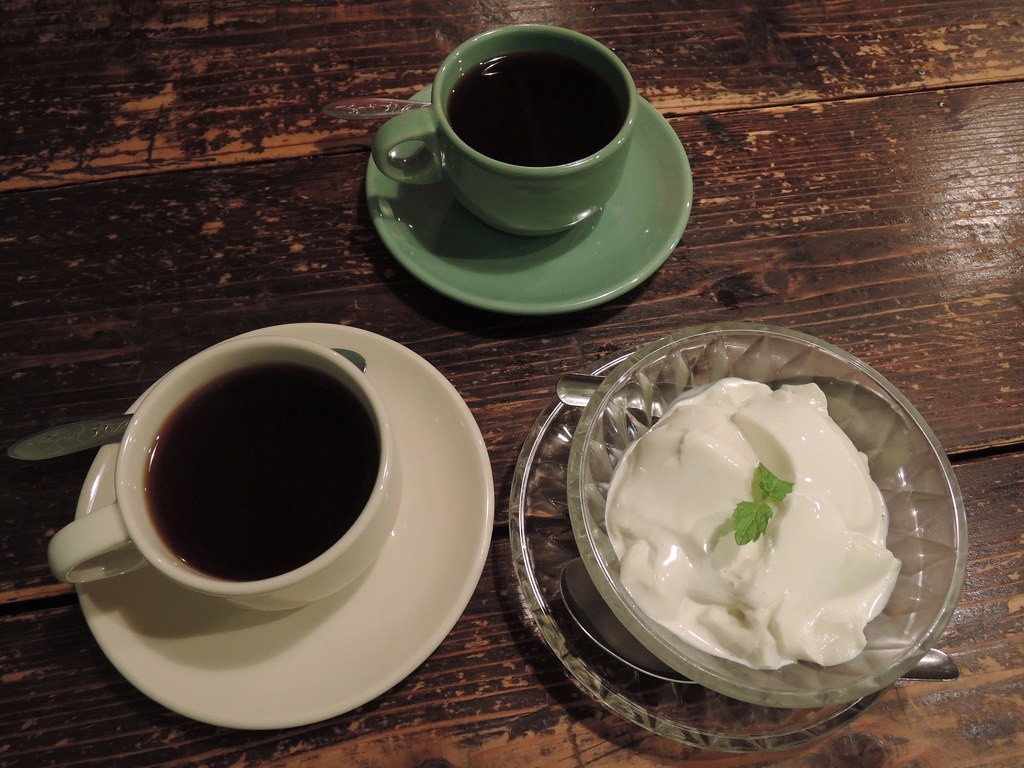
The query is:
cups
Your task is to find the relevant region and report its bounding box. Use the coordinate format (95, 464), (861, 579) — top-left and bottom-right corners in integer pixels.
(47, 336), (402, 611)
(373, 25), (637, 238)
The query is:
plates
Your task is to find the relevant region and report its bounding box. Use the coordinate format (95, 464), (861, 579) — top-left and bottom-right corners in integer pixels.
(365, 86), (696, 313)
(508, 345), (925, 754)
(75, 323), (495, 730)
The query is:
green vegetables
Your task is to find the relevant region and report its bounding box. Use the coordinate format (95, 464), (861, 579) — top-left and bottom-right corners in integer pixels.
(730, 462), (795, 545)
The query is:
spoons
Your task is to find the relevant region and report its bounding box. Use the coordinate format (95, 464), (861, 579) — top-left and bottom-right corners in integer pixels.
(560, 549), (959, 684)
(6, 348), (366, 459)
(556, 373), (913, 471)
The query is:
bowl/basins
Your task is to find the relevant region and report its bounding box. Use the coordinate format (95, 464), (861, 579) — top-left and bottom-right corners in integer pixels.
(566, 321), (968, 708)
